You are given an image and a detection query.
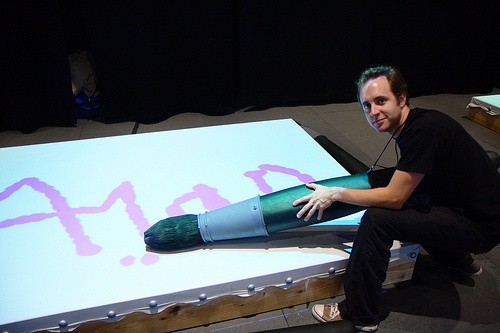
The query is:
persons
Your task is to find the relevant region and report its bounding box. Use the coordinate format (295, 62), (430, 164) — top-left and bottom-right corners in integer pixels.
(292, 67), (500, 333)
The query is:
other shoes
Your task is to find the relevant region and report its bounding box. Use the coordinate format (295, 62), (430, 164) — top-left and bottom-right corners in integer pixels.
(311, 302), (377, 331)
(469, 257), (484, 276)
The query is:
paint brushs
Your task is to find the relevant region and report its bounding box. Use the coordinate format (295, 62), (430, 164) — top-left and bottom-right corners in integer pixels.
(143, 167), (396, 252)
